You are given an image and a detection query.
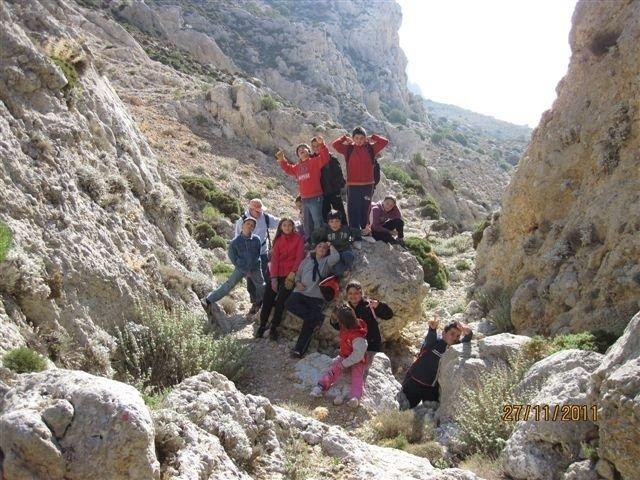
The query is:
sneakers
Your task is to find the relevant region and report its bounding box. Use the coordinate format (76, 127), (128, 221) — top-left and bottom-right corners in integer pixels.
(256, 327), (263, 337)
(361, 229), (369, 236)
(310, 385), (322, 399)
(347, 398), (357, 407)
(269, 328), (277, 339)
(291, 350), (302, 358)
(200, 298), (210, 309)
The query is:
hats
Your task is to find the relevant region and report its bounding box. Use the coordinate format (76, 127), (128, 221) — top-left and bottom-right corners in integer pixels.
(249, 198), (262, 212)
(242, 218), (256, 228)
(328, 210), (340, 219)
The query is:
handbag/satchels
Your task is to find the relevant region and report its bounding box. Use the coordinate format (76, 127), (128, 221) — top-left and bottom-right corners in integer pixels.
(320, 275), (339, 302)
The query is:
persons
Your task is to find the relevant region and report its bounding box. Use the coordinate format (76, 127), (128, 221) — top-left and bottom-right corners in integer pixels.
(199, 217), (268, 309)
(235, 198), (282, 315)
(310, 303), (368, 409)
(369, 195), (404, 245)
(282, 240), (340, 357)
(274, 125), (389, 283)
(330, 282), (394, 353)
(255, 217), (303, 340)
(397, 316), (472, 407)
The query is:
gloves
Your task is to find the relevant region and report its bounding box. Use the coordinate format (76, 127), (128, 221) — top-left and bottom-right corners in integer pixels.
(271, 278), (278, 293)
(284, 273), (295, 291)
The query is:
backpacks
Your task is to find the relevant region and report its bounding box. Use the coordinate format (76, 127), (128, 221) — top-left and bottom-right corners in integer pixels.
(346, 143), (380, 190)
